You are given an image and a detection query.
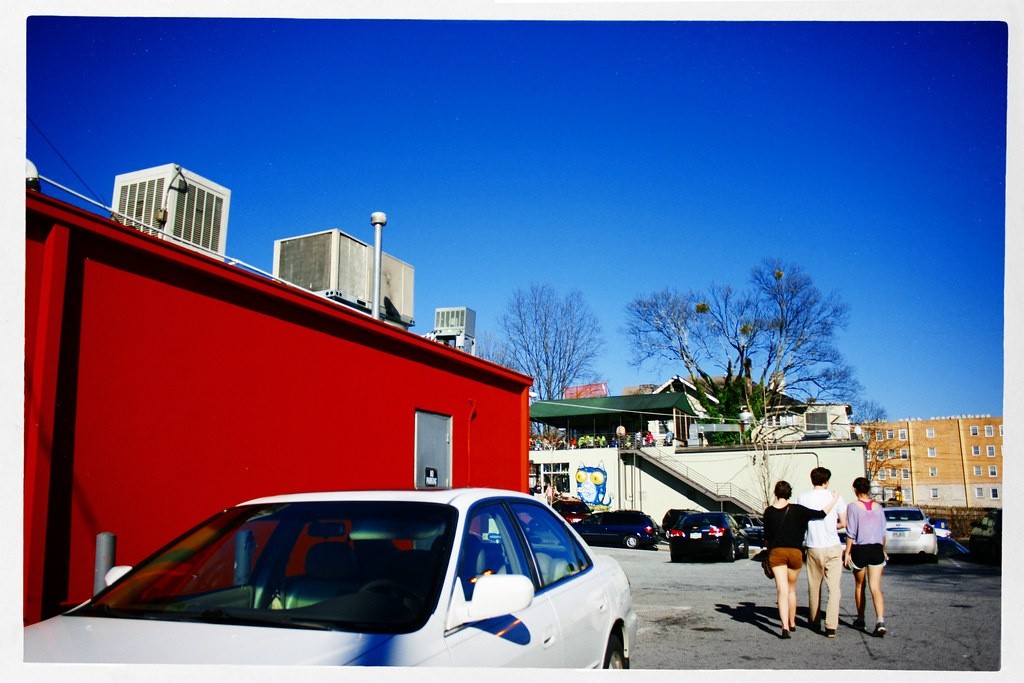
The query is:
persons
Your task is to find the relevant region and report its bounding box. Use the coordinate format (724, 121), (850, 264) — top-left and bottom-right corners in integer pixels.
(529, 432), (657, 449)
(665, 431), (673, 443)
(544, 478), (560, 505)
(843, 477), (889, 638)
(534, 479), (542, 493)
(896, 487), (903, 504)
(798, 467), (847, 637)
(763, 481), (839, 638)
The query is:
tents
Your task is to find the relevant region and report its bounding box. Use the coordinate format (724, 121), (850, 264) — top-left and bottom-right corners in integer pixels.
(529, 391), (698, 426)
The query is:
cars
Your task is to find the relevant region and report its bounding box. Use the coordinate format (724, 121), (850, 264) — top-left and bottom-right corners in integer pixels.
(24, 487), (639, 669)
(880, 507), (938, 558)
(663, 508), (768, 562)
(552, 499), (660, 549)
(970, 509), (1002, 565)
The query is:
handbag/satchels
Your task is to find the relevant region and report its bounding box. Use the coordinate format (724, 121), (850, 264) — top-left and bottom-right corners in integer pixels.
(761, 548), (775, 579)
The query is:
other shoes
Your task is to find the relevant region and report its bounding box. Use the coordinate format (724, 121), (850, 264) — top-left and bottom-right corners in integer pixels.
(790, 626), (796, 631)
(875, 621), (886, 636)
(824, 627), (836, 638)
(853, 619), (865, 630)
(782, 629), (791, 639)
(809, 617), (821, 630)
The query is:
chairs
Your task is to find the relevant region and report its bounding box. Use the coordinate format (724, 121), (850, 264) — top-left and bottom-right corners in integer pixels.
(284, 541), (352, 609)
(420, 528), (486, 612)
